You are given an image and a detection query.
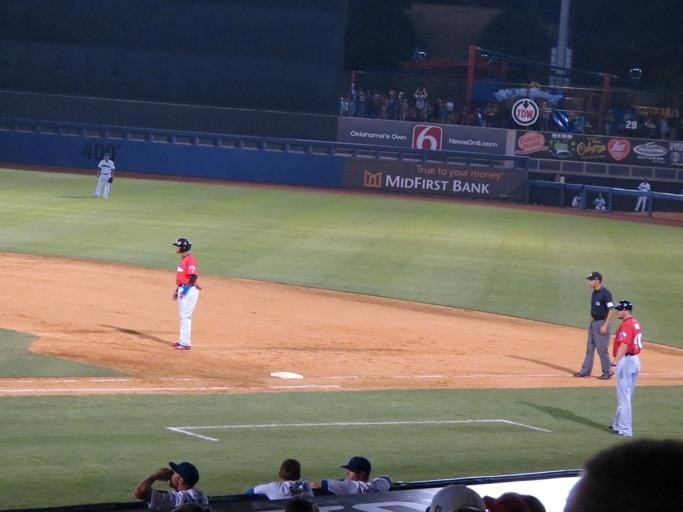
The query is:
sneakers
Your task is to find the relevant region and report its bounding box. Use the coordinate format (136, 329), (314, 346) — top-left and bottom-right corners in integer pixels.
(575, 372), (591, 376)
(174, 343), (191, 349)
(608, 426), (632, 435)
(600, 371), (614, 379)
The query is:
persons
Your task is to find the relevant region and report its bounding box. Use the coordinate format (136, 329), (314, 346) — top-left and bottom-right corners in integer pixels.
(522, 497), (545, 512)
(245, 459), (314, 500)
(570, 194), (583, 208)
(574, 272), (612, 380)
(94, 153), (115, 199)
(562, 438), (682, 512)
(173, 237), (202, 350)
(481, 492), (525, 511)
(604, 107), (683, 139)
(634, 179), (650, 213)
(133, 461), (208, 510)
(339, 87), (496, 128)
(310, 456), (391, 496)
(607, 300), (641, 436)
(591, 191), (606, 212)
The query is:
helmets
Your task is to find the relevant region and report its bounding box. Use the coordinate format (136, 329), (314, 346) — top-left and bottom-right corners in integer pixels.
(614, 301), (632, 310)
(174, 238), (191, 251)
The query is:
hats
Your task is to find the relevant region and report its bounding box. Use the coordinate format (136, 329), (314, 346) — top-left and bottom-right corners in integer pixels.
(169, 462), (198, 483)
(586, 272), (602, 280)
(341, 457), (371, 473)
